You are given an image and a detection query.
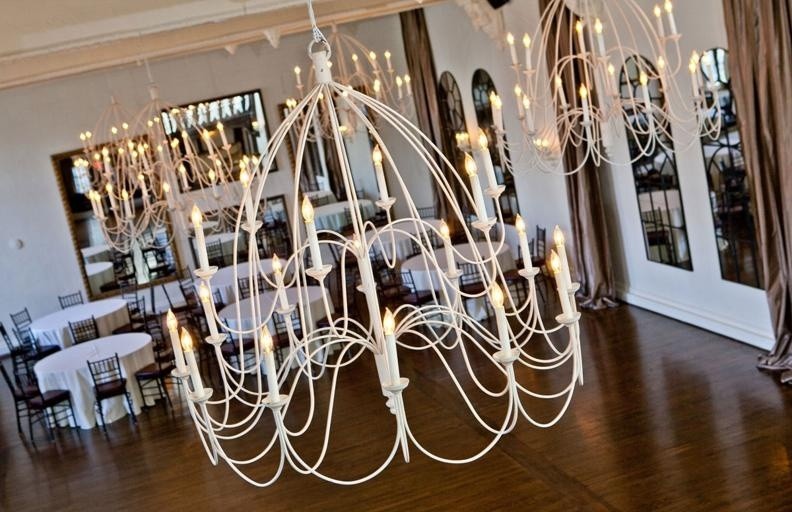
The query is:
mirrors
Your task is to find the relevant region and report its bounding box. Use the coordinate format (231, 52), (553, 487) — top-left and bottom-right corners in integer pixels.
(692, 45), (766, 291)
